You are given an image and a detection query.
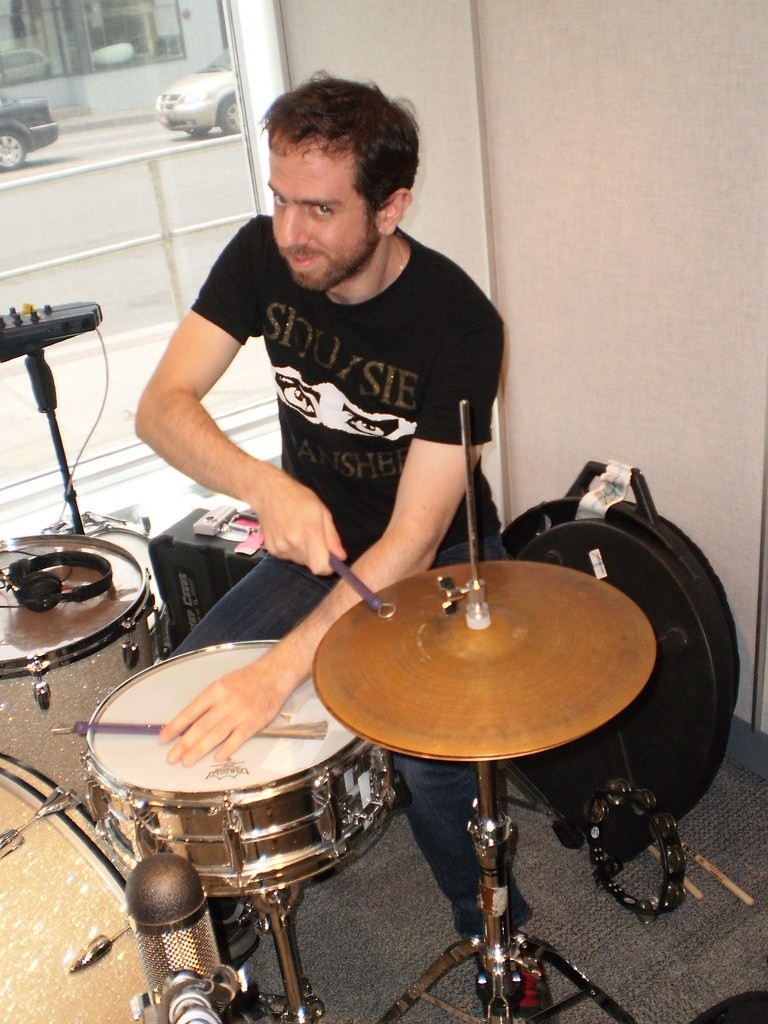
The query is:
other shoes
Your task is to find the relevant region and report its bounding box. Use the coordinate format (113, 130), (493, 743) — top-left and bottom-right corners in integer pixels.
(218, 909), (258, 959)
(476, 949), (559, 1024)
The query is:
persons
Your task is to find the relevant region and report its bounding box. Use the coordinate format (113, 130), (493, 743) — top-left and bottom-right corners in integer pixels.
(134, 77), (555, 1024)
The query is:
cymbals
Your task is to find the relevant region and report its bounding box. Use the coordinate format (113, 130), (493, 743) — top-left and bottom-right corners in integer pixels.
(582, 777), (688, 926)
(313, 558), (659, 763)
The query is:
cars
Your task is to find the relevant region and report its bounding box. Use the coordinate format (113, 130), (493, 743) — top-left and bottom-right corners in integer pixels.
(0, 48), (53, 85)
(0, 88), (61, 175)
(153, 46), (243, 137)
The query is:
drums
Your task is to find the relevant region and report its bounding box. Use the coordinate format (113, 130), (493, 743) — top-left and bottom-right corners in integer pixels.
(85, 527), (170, 667)
(0, 534), (155, 804)
(80, 639), (396, 897)
(0, 752), (157, 1024)
